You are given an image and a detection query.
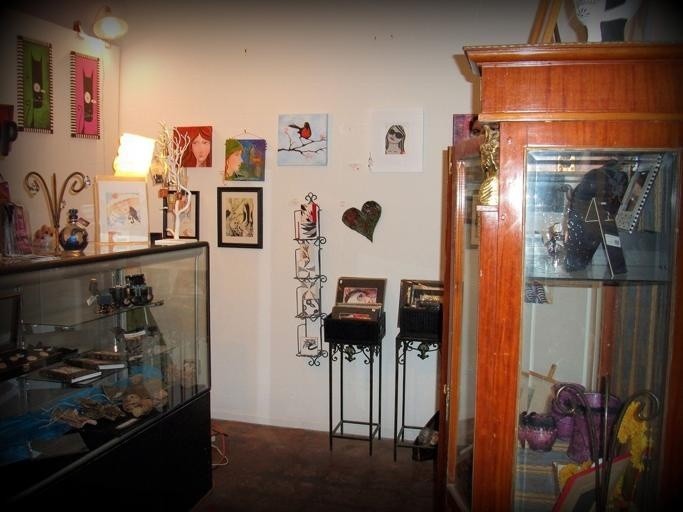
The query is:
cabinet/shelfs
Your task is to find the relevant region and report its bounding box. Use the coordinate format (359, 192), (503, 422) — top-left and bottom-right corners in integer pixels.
(0, 237), (216, 512)
(434, 40), (682, 512)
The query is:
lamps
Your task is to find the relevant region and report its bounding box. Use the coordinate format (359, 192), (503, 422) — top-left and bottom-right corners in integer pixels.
(71, 3), (131, 50)
(111, 114), (202, 247)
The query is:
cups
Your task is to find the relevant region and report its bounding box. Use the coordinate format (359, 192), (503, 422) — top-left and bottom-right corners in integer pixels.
(93, 272), (154, 316)
(525, 413), (559, 451)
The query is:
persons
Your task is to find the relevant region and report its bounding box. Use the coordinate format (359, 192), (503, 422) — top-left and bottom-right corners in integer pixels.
(225, 139), (244, 180)
(385, 123), (406, 154)
(187, 127), (211, 167)
(468, 116), (485, 138)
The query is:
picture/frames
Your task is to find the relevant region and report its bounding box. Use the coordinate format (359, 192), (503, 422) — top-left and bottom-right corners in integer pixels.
(91, 174), (151, 248)
(70, 50), (101, 140)
(216, 185), (264, 250)
(162, 190), (199, 241)
(16, 34), (54, 136)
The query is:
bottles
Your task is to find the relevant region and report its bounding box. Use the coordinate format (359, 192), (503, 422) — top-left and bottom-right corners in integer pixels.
(59, 208), (88, 251)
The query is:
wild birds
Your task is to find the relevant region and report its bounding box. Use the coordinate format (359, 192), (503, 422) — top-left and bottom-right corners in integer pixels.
(288, 122), (314, 146)
